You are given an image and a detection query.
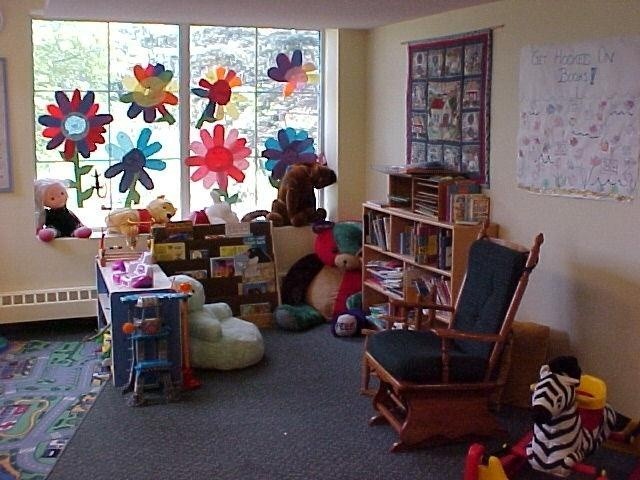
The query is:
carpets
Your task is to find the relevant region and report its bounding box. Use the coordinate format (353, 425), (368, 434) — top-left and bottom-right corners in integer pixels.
(0, 334), (109, 475)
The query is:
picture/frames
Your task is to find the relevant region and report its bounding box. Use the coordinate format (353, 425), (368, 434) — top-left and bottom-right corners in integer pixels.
(0, 56), (14, 193)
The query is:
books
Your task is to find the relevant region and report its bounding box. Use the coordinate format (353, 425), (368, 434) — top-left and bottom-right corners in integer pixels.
(153, 221), (275, 328)
(366, 165), (489, 333)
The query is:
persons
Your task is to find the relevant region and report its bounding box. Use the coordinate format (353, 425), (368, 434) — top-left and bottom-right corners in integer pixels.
(33, 178), (93, 243)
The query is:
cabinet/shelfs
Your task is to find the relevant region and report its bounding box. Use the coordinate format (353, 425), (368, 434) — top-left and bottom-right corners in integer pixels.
(359, 169), (500, 340)
(93, 219), (280, 411)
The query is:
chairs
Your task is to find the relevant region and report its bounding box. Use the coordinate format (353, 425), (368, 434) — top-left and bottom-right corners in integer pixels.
(359, 221), (544, 455)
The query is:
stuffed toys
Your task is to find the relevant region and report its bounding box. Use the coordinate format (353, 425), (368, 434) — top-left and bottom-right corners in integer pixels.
(265, 162), (337, 223)
(274, 218), (366, 339)
(188, 190), (240, 224)
(169, 275), (267, 371)
(105, 193), (178, 235)
